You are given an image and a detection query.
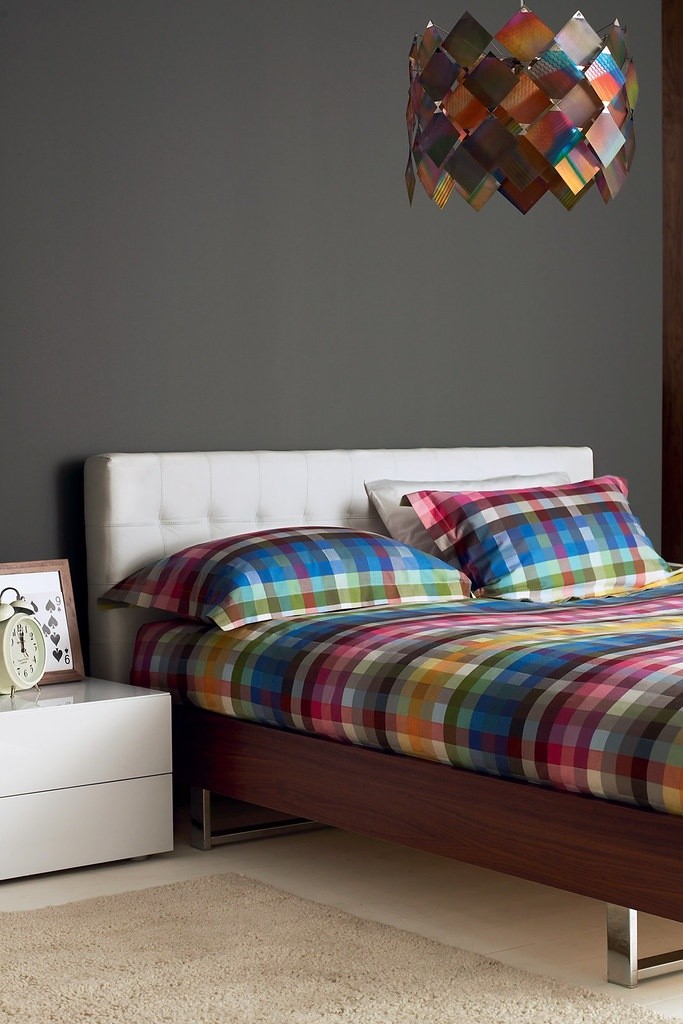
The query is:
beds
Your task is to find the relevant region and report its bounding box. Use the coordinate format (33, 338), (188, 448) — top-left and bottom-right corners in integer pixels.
(85, 448), (683, 987)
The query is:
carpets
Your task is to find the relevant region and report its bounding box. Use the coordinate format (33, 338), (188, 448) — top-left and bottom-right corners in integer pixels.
(0, 872), (683, 1024)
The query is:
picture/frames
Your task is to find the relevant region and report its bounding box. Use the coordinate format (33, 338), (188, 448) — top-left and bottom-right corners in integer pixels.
(0, 558), (86, 686)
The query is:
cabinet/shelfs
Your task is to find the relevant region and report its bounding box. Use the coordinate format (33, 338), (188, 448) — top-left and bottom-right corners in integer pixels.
(0, 676), (174, 880)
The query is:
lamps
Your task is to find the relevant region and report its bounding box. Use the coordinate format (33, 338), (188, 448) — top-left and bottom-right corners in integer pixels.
(405, 0), (639, 215)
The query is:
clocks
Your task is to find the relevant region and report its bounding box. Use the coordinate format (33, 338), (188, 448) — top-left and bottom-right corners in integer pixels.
(0, 588), (47, 698)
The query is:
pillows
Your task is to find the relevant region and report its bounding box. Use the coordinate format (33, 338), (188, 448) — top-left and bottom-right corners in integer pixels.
(96, 525), (473, 633)
(363, 472), (683, 604)
